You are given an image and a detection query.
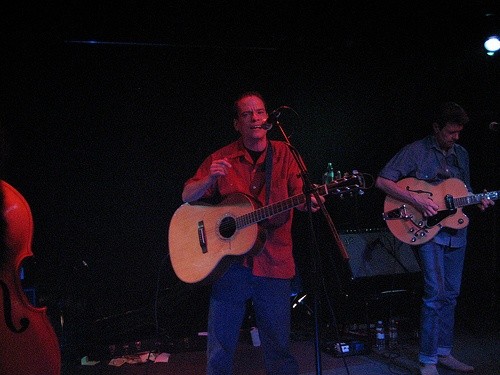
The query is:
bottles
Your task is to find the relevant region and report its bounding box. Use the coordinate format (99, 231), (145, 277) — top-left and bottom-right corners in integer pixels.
(375, 320), (385, 349)
(388, 320), (398, 347)
(325, 163), (334, 184)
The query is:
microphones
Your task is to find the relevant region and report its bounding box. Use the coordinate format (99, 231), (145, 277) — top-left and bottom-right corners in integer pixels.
(262, 106), (282, 130)
(489, 122), (500, 130)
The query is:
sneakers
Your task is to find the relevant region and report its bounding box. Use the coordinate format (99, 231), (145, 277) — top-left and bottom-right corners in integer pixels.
(439, 354), (474, 372)
(417, 361), (440, 375)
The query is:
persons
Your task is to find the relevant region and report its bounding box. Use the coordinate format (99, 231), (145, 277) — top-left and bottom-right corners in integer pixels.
(181, 89), (326, 375)
(375, 103), (495, 375)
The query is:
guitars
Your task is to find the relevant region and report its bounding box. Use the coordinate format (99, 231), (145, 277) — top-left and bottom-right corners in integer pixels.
(169, 169), (367, 284)
(384, 178), (500, 245)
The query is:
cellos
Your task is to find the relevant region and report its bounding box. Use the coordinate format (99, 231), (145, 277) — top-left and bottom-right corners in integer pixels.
(0, 180), (61, 375)
